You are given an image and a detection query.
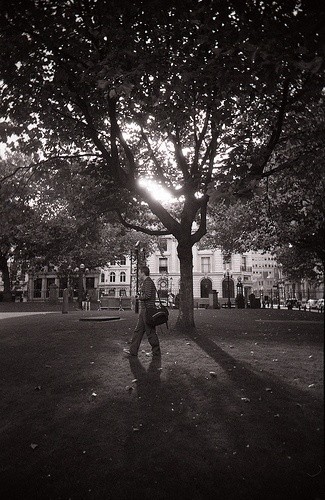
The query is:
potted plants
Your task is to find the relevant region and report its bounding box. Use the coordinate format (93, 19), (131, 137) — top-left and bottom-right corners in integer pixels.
(234, 293), (262, 309)
(286, 302), (294, 310)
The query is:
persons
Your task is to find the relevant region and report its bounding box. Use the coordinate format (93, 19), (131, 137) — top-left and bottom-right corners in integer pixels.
(76, 289), (91, 309)
(123, 265), (162, 358)
(264, 295), (324, 313)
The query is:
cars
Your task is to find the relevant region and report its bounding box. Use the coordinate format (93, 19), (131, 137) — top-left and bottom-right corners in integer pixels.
(258, 294), (324, 311)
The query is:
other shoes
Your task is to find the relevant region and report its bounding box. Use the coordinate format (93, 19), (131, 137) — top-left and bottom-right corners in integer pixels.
(145, 351), (159, 357)
(123, 349), (135, 356)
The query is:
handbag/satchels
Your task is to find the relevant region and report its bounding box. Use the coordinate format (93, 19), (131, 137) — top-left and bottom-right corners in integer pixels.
(146, 304), (168, 326)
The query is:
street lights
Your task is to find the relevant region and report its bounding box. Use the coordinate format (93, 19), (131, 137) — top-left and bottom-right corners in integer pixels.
(134, 240), (141, 314)
(223, 267), (233, 310)
(141, 247), (148, 267)
(74, 263), (90, 309)
(273, 275), (282, 310)
